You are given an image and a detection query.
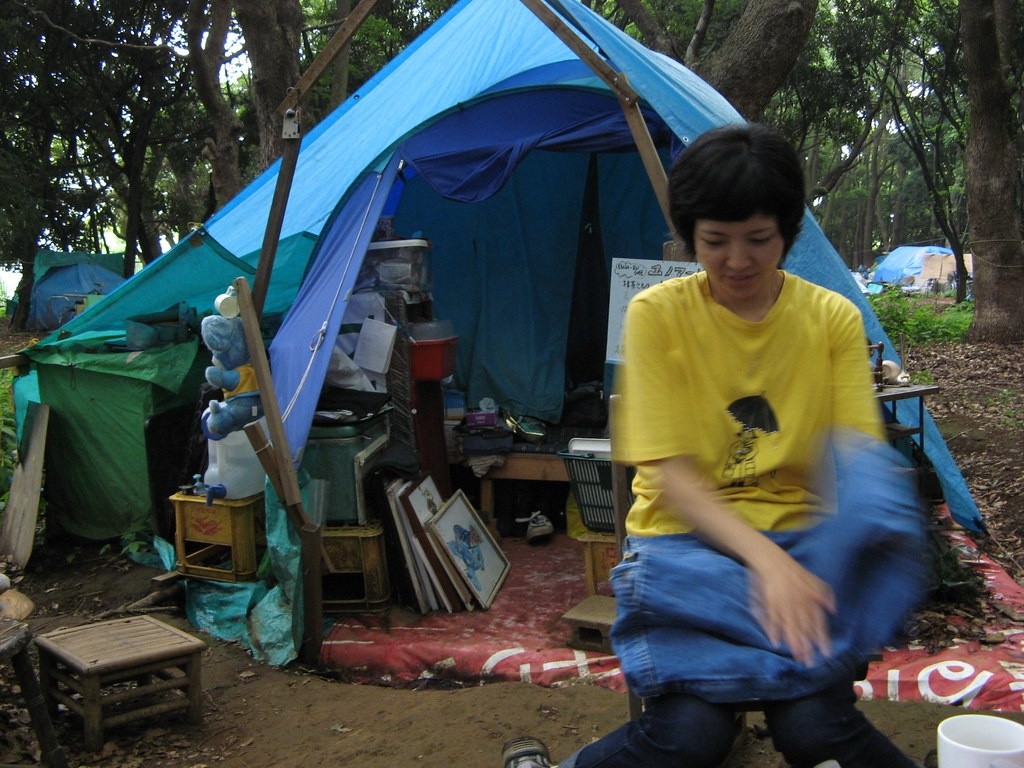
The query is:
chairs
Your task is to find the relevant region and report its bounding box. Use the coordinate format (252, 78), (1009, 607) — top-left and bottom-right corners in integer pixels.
(609, 394), (884, 768)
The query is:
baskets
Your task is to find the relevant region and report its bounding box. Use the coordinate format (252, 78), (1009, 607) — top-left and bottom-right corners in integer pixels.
(561, 449), (634, 533)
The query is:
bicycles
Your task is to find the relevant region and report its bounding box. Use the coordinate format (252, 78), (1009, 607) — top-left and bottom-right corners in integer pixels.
(943, 270), (973, 293)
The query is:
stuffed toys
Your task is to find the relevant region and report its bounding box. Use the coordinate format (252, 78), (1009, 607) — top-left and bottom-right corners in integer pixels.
(201, 315), (265, 434)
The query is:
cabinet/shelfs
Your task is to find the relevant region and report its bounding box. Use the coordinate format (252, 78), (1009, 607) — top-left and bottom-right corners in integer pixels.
(873, 383), (941, 526)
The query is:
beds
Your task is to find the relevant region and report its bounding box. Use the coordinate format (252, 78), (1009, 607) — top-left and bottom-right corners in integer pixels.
(441, 423), (619, 532)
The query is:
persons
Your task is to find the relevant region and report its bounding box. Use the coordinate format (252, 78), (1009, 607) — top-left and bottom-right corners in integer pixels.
(501, 124), (917, 768)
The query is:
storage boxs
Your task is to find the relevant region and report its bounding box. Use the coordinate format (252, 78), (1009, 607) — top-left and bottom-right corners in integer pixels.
(408, 320), (459, 382)
(352, 238), (429, 292)
(320, 509), (391, 614)
(293, 414), (390, 524)
(169, 489), (268, 584)
(556, 438), (636, 595)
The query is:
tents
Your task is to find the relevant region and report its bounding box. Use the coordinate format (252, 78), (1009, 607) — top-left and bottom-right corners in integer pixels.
(25, 263), (127, 331)
(872, 246), (953, 289)
(18, 0), (985, 512)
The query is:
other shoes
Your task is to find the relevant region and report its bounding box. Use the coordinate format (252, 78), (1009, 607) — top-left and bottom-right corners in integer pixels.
(518, 510), (554, 541)
(501, 737), (550, 768)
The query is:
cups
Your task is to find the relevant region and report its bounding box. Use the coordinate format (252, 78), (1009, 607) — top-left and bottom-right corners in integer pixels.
(936, 713), (1024, 768)
(214, 286), (240, 319)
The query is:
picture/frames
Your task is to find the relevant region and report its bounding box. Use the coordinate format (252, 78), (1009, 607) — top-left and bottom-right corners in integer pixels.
(386, 472), (511, 615)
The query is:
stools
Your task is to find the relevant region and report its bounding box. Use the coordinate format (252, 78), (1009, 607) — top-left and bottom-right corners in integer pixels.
(31, 615), (208, 753)
